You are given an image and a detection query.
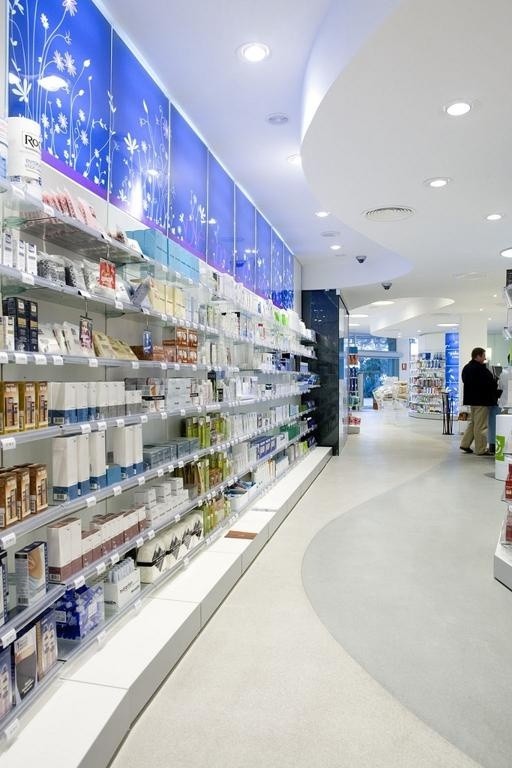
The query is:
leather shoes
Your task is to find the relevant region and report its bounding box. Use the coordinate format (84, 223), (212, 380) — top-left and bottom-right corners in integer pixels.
(460, 446), (494, 456)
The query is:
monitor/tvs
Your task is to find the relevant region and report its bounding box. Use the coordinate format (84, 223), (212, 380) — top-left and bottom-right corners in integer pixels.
(492, 366), (502, 378)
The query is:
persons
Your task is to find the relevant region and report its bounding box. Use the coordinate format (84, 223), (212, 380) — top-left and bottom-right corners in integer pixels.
(457, 345), (500, 457)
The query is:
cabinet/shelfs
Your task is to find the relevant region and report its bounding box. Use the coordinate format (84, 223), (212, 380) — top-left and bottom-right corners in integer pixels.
(490, 268), (512, 592)
(0, 178), (361, 767)
(409, 357), (445, 421)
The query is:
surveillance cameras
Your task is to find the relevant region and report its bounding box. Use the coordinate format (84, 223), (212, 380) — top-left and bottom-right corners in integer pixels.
(356, 256), (366, 263)
(382, 283), (392, 289)
(231, 260), (245, 268)
